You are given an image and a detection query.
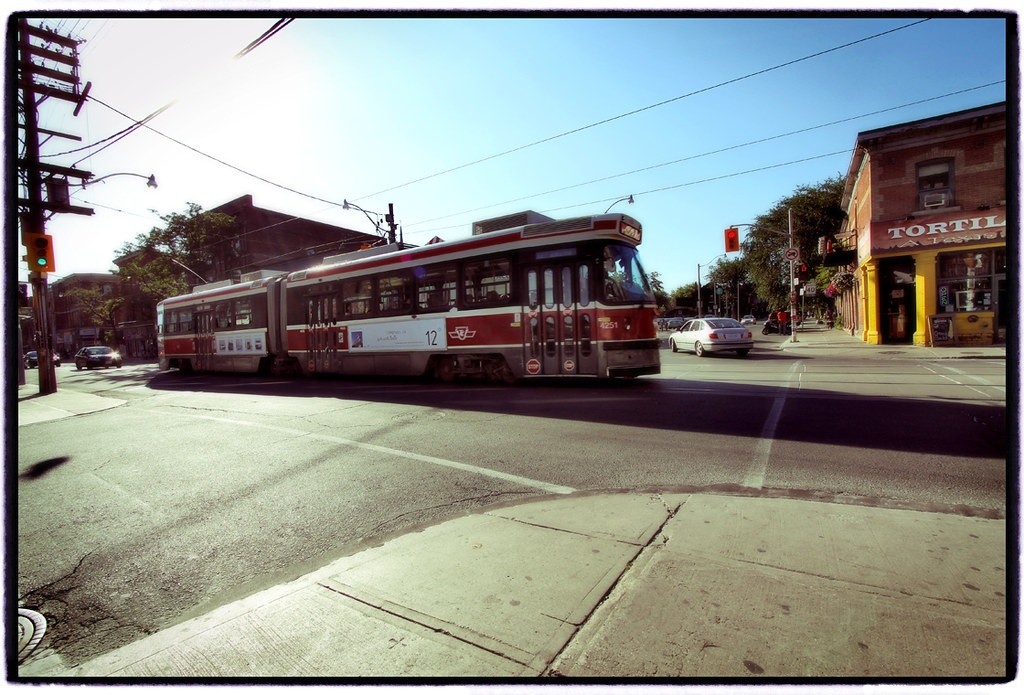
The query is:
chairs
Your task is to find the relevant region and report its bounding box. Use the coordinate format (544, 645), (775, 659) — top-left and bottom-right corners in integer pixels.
(714, 322), (733, 328)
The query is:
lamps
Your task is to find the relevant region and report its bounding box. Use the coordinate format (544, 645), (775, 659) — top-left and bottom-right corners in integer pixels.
(906, 215), (914, 220)
(978, 205), (990, 211)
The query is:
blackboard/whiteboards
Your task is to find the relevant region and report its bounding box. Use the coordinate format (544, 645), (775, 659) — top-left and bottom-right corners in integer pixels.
(928, 314), (957, 342)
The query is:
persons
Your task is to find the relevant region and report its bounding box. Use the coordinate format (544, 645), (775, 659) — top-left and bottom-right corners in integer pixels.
(826, 306), (833, 329)
(777, 308), (787, 336)
(971, 281), (985, 310)
(467, 287), (499, 303)
(766, 304), (770, 318)
(345, 281), (449, 317)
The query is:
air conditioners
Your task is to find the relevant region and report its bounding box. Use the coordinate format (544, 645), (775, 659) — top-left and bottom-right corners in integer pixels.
(924, 194), (949, 208)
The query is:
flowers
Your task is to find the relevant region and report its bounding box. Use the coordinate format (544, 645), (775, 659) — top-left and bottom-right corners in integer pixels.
(823, 272), (853, 299)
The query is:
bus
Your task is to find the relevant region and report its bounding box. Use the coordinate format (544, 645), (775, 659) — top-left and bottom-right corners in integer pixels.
(155, 210), (663, 389)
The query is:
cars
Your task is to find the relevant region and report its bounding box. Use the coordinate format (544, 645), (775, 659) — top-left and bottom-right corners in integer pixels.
(23, 350), (61, 369)
(668, 317), (694, 329)
(740, 315), (756, 326)
(668, 317), (755, 357)
(768, 309), (807, 327)
(74, 346), (122, 370)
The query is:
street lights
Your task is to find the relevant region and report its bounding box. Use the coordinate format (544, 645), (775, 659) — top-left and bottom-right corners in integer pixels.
(29, 173), (158, 395)
(343, 199), (395, 245)
(698, 253), (727, 319)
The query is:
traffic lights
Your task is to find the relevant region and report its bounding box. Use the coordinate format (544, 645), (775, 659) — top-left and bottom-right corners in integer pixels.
(27, 232), (55, 272)
(725, 228), (740, 252)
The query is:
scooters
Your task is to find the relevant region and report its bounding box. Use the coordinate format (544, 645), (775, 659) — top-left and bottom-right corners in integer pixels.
(762, 317), (792, 335)
